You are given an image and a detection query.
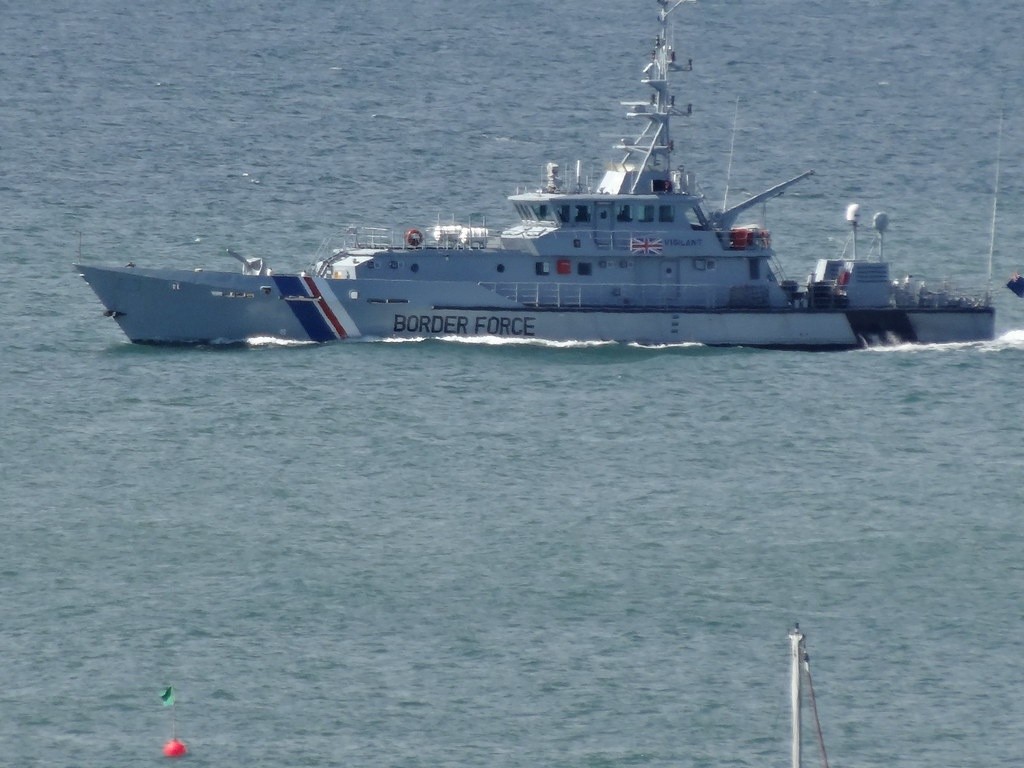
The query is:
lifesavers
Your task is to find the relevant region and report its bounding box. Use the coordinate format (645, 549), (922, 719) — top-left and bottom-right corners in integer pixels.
(406, 229), (424, 247)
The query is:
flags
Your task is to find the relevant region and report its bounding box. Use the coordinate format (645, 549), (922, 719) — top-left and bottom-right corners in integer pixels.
(160, 686), (174, 708)
(1006, 273), (1024, 298)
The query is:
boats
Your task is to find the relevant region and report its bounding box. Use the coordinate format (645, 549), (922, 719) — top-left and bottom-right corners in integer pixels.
(75, 1), (996, 351)
